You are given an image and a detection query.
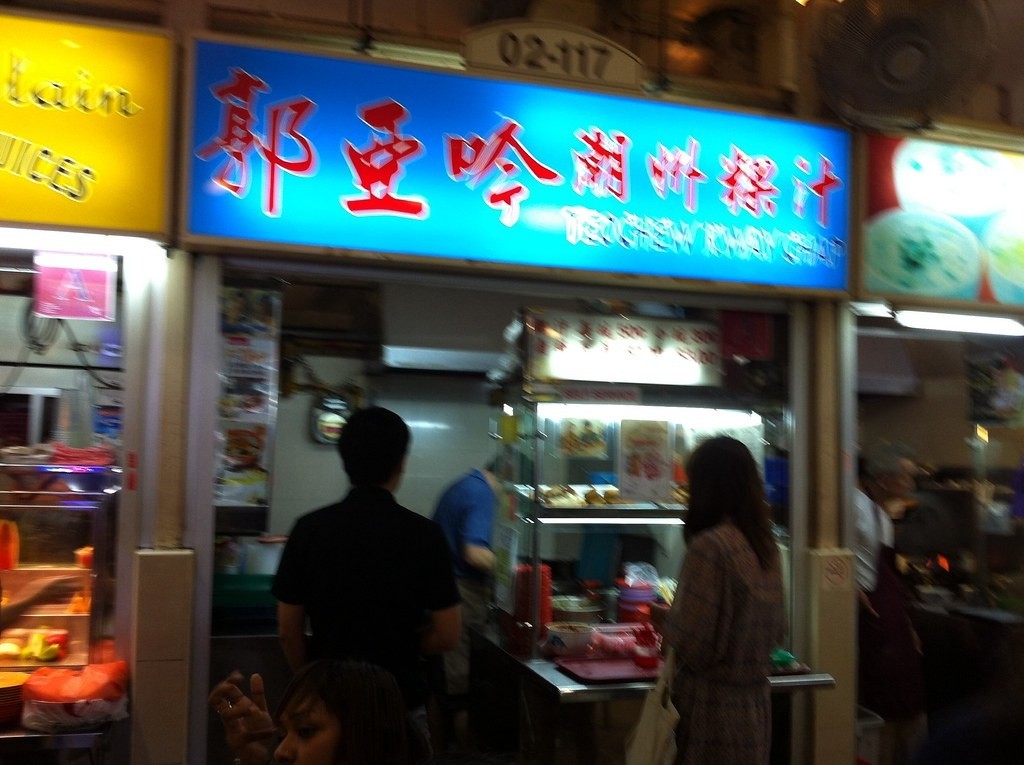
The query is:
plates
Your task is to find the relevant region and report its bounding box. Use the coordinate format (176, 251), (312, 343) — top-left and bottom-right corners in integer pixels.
(0, 672), (29, 720)
(511, 566), (551, 640)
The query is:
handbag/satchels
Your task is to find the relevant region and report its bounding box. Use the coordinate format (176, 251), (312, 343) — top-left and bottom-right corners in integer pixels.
(621, 647), (683, 765)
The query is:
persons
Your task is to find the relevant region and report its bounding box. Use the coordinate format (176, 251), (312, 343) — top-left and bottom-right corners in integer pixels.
(271, 405), (465, 765)
(432, 443), (536, 756)
(616, 434), (793, 765)
(768, 448), (1024, 765)
(207, 659), (409, 765)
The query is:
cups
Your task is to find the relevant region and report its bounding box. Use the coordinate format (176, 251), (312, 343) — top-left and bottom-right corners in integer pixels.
(49, 389), (94, 449)
(635, 642), (658, 669)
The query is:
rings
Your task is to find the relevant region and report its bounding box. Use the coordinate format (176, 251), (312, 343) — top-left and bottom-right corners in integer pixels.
(218, 701), (232, 714)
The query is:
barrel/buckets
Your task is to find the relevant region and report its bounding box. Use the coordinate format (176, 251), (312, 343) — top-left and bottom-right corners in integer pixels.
(618, 587), (655, 624)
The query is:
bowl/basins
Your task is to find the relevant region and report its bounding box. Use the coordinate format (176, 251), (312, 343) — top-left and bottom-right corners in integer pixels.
(987, 207), (1023, 307)
(867, 210), (982, 301)
(32, 699), (102, 725)
(546, 624), (595, 657)
(224, 429), (264, 465)
(551, 597), (608, 623)
